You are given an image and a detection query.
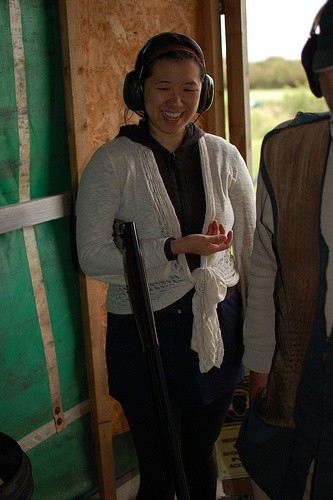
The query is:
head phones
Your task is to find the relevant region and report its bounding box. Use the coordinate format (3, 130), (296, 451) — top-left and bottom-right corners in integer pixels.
(123, 32), (215, 114)
(301, 0), (333, 98)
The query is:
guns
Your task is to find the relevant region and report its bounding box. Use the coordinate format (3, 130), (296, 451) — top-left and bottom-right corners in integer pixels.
(70, 215), (189, 500)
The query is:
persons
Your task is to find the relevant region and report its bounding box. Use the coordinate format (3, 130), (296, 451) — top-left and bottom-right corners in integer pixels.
(243, 0), (333, 500)
(77, 31), (257, 500)
